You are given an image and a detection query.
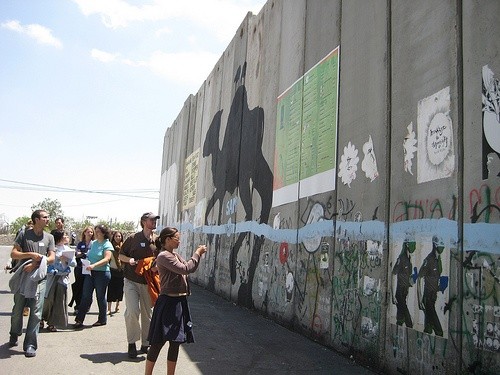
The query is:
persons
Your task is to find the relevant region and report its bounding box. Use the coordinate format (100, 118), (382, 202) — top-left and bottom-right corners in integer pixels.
(145, 227), (207, 375)
(48, 217), (68, 247)
(11, 219), (34, 316)
(72, 225), (115, 330)
(8, 210), (56, 357)
(39, 230), (78, 332)
(118, 212), (160, 358)
(107, 231), (124, 316)
(73, 226), (96, 318)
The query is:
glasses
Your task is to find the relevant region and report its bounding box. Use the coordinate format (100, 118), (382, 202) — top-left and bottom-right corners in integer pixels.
(37, 216), (48, 219)
(172, 237), (180, 241)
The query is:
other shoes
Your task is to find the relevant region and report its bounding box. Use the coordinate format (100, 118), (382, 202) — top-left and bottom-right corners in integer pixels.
(137, 349), (148, 354)
(128, 347), (137, 357)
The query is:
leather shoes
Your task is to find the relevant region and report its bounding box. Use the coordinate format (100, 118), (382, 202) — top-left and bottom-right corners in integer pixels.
(10, 336), (18, 346)
(26, 344), (36, 356)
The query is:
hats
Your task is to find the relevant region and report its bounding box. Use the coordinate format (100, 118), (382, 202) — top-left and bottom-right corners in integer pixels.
(141, 212), (160, 219)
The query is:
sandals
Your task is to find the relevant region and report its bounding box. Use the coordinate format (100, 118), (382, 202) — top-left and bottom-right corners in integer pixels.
(47, 325), (57, 332)
(40, 321), (44, 330)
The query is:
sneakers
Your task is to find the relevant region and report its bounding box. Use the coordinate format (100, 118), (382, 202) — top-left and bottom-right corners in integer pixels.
(72, 322), (84, 328)
(92, 322), (107, 326)
(23, 307), (29, 316)
(115, 309), (120, 313)
(106, 311), (111, 315)
(73, 310), (79, 316)
